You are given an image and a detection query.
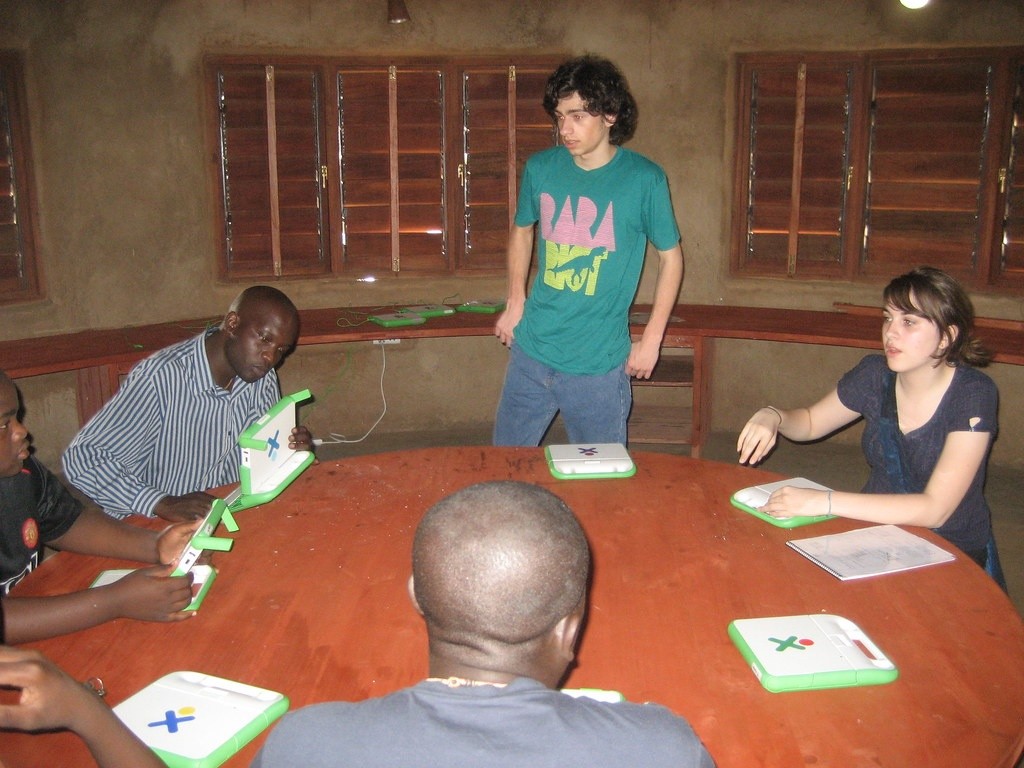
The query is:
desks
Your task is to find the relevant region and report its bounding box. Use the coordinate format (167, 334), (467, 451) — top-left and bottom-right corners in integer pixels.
(0, 446), (1024, 768)
(0, 303), (1024, 462)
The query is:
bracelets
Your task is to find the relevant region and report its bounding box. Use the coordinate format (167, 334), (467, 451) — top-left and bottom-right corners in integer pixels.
(764, 407), (782, 424)
(828, 491), (831, 514)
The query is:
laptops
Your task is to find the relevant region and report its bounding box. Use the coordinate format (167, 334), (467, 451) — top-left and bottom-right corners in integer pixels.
(368, 299), (506, 328)
(106, 669), (289, 768)
(729, 476), (839, 529)
(88, 392), (314, 612)
(726, 613), (900, 693)
(544, 443), (638, 481)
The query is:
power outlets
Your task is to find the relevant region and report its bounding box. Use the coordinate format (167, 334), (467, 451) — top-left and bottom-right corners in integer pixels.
(373, 338), (403, 346)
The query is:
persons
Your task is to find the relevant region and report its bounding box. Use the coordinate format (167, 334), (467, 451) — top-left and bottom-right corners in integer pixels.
(250, 481), (717, 768)
(0, 372), (215, 644)
(61, 286), (321, 524)
(492, 53), (685, 446)
(0, 644), (169, 768)
(736, 265), (1008, 593)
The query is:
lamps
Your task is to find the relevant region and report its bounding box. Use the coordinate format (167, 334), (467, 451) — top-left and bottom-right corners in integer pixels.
(387, 0), (411, 26)
